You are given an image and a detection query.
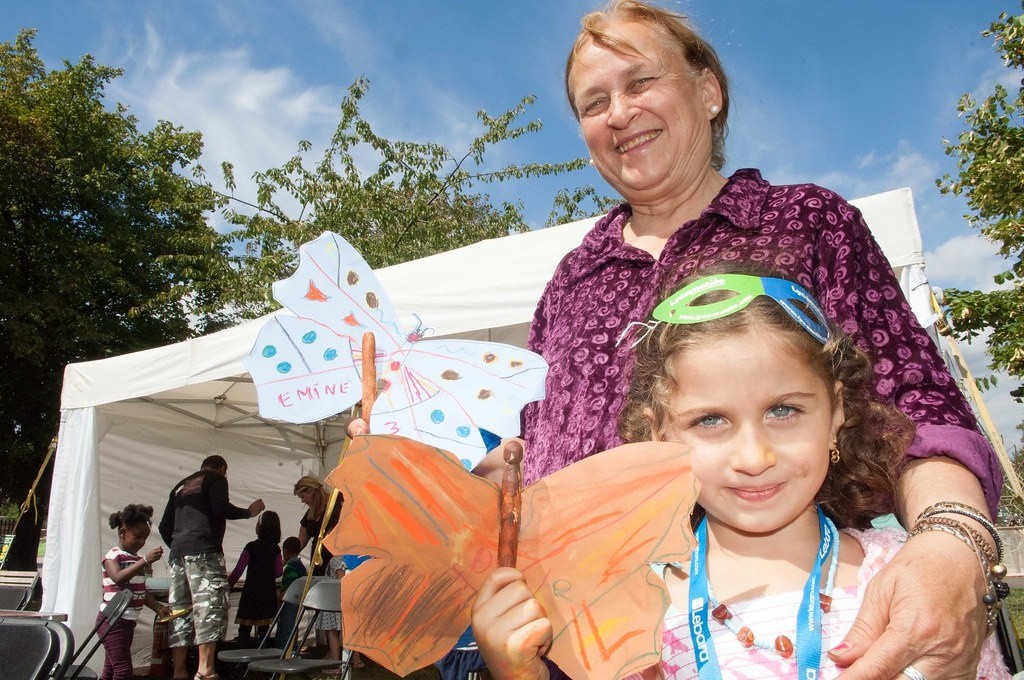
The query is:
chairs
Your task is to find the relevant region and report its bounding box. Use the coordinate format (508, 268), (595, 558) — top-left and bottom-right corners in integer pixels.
(248, 578), (354, 680)
(217, 575), (332, 680)
(0, 571), (134, 680)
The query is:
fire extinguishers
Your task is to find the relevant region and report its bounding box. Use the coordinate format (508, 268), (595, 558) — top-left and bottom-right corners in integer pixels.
(149, 614), (171, 677)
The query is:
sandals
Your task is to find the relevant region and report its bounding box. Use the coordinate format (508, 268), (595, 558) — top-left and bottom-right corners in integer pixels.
(194, 672), (221, 680)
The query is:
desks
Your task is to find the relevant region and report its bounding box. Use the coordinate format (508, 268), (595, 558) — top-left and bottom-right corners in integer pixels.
(0, 609), (68, 621)
(147, 580), (245, 604)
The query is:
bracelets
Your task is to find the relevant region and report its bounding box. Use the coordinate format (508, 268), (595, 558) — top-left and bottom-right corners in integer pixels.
(907, 502), (1010, 638)
(143, 555), (151, 566)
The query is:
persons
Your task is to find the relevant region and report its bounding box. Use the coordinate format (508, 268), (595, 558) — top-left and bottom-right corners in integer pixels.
(96, 427), (528, 680)
(470, 265), (1011, 680)
(346, 0), (1010, 680)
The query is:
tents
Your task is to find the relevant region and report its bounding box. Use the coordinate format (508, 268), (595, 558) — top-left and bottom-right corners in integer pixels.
(39, 186), (941, 680)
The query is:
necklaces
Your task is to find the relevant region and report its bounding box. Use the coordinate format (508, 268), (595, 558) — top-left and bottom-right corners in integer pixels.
(706, 517), (840, 657)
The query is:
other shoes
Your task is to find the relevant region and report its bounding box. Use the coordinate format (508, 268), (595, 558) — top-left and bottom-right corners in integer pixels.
(322, 661), (364, 674)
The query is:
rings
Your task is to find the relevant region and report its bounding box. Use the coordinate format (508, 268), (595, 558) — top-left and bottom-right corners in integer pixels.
(901, 665), (928, 680)
